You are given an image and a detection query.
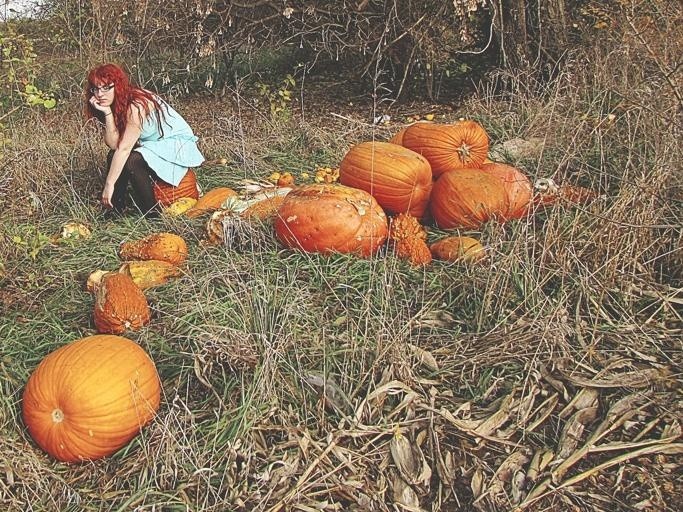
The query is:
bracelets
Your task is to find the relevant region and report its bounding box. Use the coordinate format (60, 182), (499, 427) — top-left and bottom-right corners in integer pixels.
(104, 111), (113, 117)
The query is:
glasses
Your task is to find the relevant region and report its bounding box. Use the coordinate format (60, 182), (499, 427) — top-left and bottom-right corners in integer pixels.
(89, 85), (116, 93)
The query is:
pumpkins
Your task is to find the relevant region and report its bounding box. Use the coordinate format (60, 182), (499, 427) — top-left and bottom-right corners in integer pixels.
(242, 120), (599, 265)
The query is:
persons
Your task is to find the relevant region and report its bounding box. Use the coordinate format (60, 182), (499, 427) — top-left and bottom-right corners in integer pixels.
(86, 63), (206, 229)
